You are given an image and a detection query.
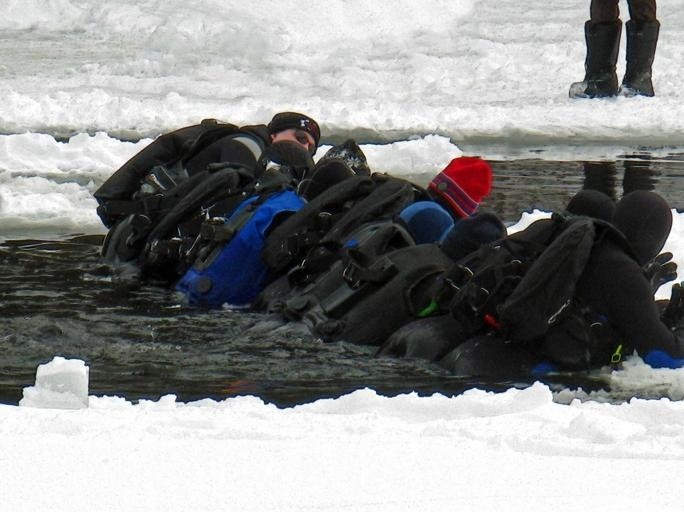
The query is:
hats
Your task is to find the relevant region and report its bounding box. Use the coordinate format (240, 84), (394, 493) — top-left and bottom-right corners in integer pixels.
(442, 212), (507, 264)
(565, 189), (614, 223)
(303, 157), (353, 202)
(400, 201), (455, 246)
(267, 112), (322, 154)
(308, 138), (371, 176)
(429, 156), (493, 218)
(611, 189), (672, 261)
(255, 140), (314, 180)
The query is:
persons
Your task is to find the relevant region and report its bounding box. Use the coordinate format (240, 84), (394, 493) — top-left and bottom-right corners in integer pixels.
(90, 106), (683, 380)
(563, 2), (658, 98)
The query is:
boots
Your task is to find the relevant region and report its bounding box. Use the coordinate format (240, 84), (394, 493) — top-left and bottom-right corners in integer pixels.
(569, 19), (661, 97)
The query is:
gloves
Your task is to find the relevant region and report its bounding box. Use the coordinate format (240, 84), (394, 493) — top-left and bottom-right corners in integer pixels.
(646, 252), (684, 334)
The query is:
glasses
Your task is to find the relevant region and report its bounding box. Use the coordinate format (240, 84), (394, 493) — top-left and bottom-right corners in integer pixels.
(294, 127), (316, 155)
